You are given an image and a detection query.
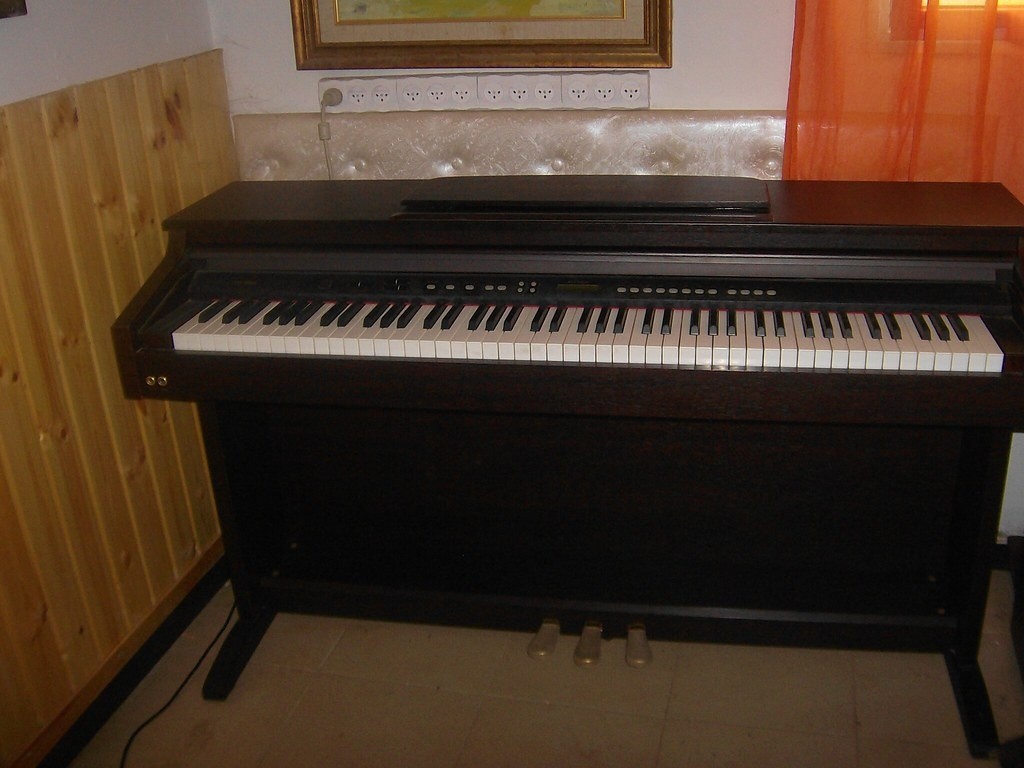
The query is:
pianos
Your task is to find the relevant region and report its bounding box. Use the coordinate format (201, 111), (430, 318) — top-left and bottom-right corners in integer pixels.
(110, 175), (1024, 757)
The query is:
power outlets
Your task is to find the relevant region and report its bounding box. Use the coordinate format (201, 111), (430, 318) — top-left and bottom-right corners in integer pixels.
(318, 70), (649, 114)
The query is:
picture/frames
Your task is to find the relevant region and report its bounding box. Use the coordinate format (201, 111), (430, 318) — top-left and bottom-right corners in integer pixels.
(290, 0), (673, 70)
